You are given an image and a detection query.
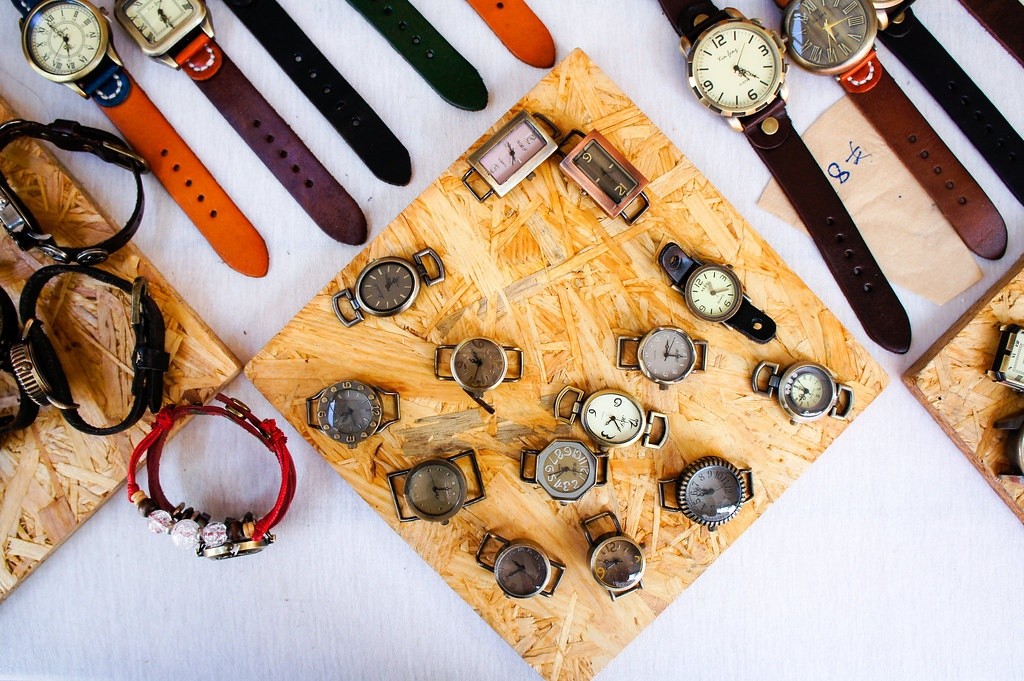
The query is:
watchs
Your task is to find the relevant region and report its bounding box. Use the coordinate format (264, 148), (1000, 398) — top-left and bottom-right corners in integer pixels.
(0, 1), (1024, 605)
